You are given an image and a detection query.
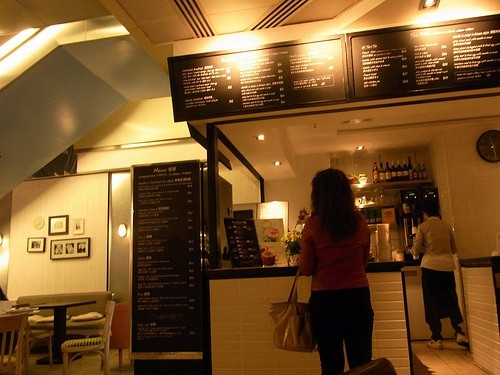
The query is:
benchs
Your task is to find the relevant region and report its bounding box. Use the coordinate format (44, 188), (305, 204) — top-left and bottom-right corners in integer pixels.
(15, 290), (113, 347)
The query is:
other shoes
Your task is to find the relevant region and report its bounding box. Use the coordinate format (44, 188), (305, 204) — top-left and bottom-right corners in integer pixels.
(456, 333), (470, 348)
(428, 339), (442, 350)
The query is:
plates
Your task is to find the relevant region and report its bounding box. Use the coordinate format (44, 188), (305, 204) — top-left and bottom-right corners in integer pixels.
(5, 307), (38, 313)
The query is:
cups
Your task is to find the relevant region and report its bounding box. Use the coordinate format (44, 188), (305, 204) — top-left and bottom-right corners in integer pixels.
(354, 195), (374, 205)
(413, 253), (419, 259)
(264, 228), (281, 242)
(358, 177), (367, 184)
(262, 254), (279, 266)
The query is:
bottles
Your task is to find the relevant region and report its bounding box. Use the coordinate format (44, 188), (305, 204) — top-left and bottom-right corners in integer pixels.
(373, 161), (428, 183)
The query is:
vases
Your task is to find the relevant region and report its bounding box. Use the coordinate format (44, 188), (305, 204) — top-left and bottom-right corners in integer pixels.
(289, 253), (301, 266)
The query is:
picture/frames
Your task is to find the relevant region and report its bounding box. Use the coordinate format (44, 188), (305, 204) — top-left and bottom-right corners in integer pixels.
(27, 237), (45, 252)
(49, 237), (91, 260)
(48, 215), (68, 235)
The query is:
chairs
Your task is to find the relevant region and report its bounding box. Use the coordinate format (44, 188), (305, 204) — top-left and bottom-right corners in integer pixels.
(0, 300), (117, 375)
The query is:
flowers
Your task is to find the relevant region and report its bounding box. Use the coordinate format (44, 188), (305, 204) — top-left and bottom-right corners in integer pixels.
(280, 228), (302, 259)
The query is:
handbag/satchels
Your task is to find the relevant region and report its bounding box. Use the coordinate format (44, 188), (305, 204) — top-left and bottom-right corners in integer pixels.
(268, 269), (316, 352)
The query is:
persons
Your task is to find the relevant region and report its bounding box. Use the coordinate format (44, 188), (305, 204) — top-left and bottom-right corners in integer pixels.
(408, 200), (469, 349)
(299, 168), (375, 375)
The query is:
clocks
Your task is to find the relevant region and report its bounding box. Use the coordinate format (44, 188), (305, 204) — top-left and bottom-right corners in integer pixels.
(476, 129), (500, 162)
